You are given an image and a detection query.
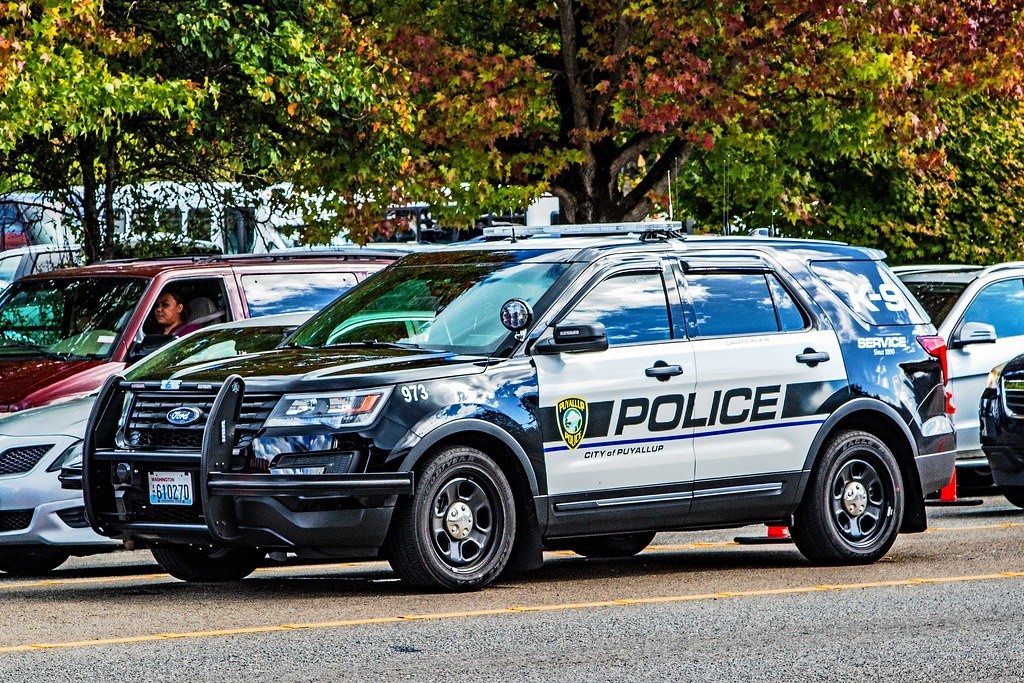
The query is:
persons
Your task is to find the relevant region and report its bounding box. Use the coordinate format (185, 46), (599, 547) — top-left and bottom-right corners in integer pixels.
(155, 291), (201, 339)
(75, 306), (99, 334)
(390, 216), (415, 243)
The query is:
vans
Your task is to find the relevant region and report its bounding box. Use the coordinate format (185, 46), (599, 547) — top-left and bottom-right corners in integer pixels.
(0, 177), (559, 423)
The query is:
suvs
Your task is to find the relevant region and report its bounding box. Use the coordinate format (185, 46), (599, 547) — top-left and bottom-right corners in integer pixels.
(78, 208), (960, 597)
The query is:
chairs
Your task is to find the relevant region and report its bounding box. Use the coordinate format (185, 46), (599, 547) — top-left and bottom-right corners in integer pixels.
(182, 298), (220, 329)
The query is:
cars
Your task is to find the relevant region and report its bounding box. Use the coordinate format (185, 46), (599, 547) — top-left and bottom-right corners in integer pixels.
(886, 261), (1024, 500)
(0, 308), (492, 579)
(979, 352), (1024, 510)
